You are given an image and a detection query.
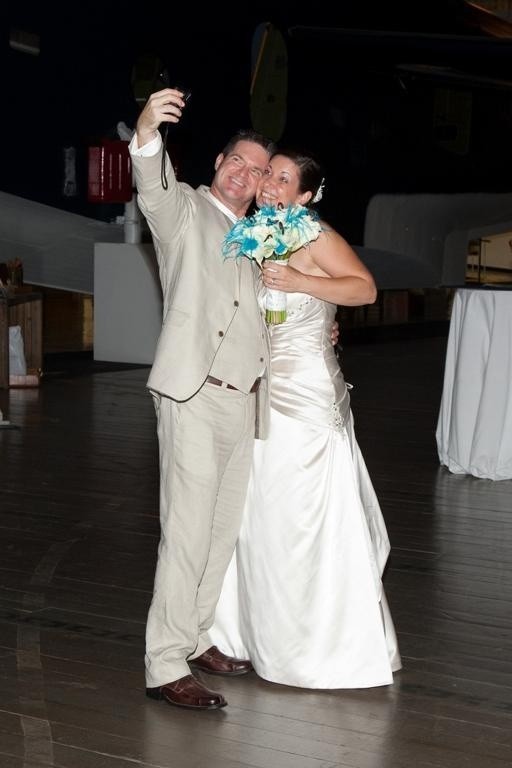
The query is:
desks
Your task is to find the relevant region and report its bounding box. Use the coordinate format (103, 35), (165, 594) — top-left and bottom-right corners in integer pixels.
(431, 287), (511, 482)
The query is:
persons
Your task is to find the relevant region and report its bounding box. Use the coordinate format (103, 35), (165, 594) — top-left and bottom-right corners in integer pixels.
(126, 88), (338, 709)
(209, 153), (404, 689)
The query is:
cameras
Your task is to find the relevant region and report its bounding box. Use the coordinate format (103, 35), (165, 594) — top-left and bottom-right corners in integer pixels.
(162, 89), (192, 127)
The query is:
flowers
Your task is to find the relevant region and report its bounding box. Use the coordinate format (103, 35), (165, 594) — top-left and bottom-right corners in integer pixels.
(220, 198), (335, 326)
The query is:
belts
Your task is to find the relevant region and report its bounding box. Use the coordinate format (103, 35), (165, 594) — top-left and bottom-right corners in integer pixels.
(206, 375), (261, 392)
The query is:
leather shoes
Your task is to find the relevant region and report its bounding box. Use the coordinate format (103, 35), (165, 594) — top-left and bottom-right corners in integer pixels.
(146, 675), (227, 710)
(187, 646), (253, 677)
